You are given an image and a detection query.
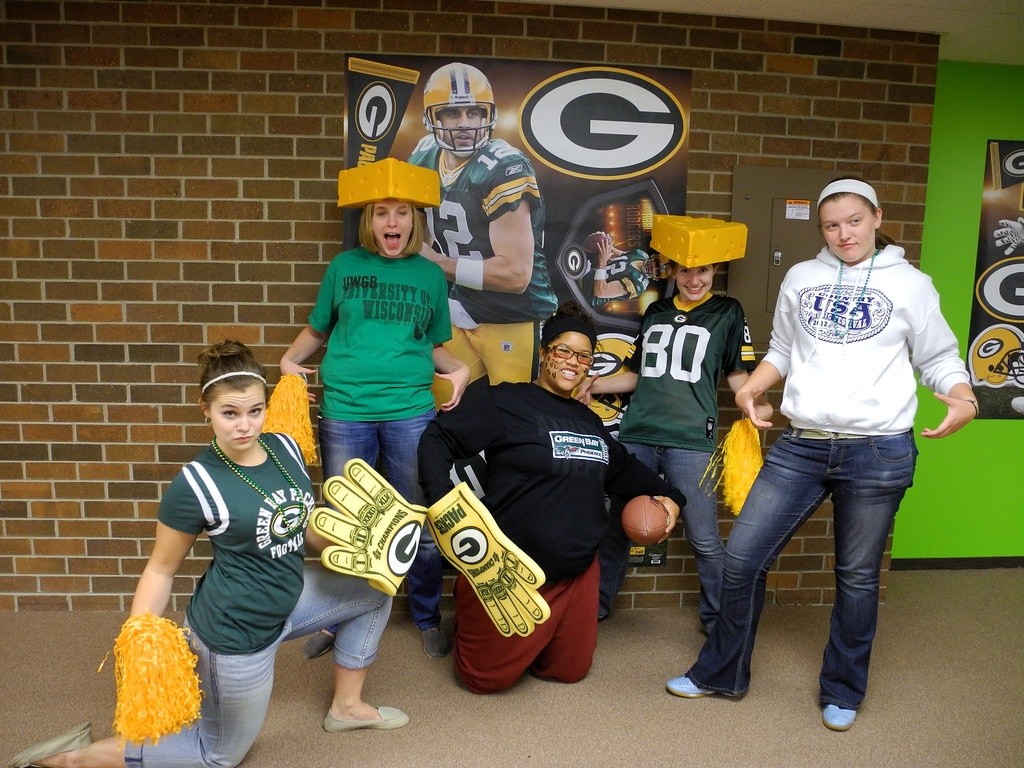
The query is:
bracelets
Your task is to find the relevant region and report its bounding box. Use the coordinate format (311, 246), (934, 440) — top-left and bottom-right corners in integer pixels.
(962, 396), (979, 421)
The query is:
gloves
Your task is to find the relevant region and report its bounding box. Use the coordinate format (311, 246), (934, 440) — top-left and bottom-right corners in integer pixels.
(993, 217), (1024, 256)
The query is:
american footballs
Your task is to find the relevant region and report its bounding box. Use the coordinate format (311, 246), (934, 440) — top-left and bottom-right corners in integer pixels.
(584, 232), (611, 253)
(622, 495), (667, 545)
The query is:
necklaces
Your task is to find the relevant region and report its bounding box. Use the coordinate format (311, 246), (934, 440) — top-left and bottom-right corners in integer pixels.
(834, 249), (879, 340)
(208, 429), (308, 535)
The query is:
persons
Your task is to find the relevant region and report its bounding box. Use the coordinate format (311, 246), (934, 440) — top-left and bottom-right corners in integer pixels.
(4, 338), (413, 768)
(662, 173), (981, 730)
(277, 185), (476, 668)
(403, 61), (558, 423)
(416, 310), (689, 696)
(573, 253), (773, 640)
(577, 231), (673, 309)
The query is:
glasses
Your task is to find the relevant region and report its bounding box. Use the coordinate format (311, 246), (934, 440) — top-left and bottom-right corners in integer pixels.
(545, 343), (597, 366)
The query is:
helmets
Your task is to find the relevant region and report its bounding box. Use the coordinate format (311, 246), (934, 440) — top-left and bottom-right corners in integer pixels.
(420, 61), (496, 144)
(651, 254), (672, 279)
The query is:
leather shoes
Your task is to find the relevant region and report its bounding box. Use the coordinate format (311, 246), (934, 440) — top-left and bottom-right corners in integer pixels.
(8, 722), (95, 768)
(323, 705), (410, 733)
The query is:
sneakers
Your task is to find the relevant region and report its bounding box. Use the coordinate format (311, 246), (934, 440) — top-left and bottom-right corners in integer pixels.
(821, 704), (857, 732)
(299, 630), (336, 659)
(666, 675), (737, 698)
(415, 623), (448, 659)
